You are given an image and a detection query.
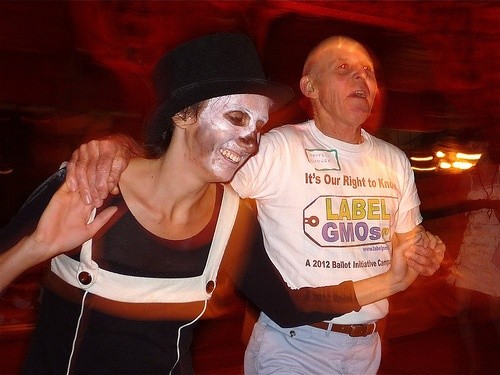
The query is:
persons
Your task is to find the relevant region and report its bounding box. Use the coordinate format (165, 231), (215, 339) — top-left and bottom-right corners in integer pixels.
(60, 36), (454, 375)
(2, 27), (443, 375)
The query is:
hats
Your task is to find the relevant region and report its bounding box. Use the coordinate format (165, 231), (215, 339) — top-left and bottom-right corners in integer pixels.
(142, 30), (274, 136)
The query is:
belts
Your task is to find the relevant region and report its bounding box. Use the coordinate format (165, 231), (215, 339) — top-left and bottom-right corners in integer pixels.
(307, 318), (388, 339)
